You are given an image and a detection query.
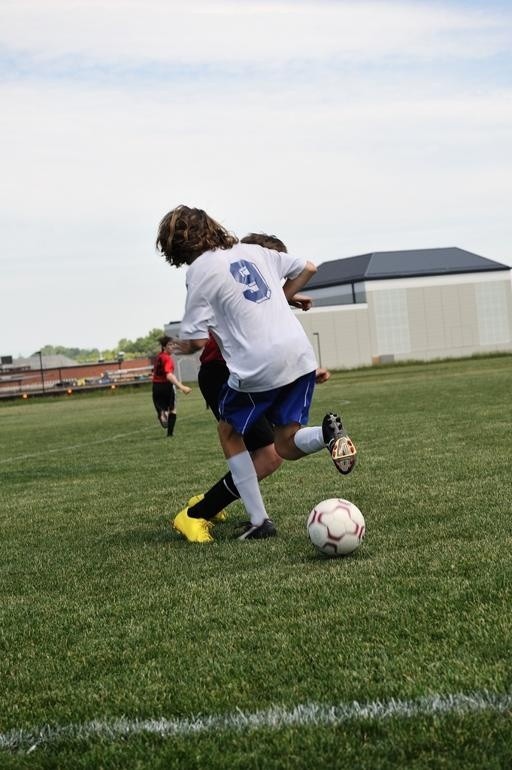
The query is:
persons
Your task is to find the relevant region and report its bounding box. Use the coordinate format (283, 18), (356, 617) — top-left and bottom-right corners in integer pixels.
(154, 203), (357, 543)
(152, 333), (193, 439)
(172, 231), (332, 544)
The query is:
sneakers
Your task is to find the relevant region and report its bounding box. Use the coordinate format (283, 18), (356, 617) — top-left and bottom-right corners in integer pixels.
(322, 413), (357, 474)
(159, 408), (169, 428)
(187, 493), (229, 522)
(232, 518), (277, 540)
(172, 506), (215, 543)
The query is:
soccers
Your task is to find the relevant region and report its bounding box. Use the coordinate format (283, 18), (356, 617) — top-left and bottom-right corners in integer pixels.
(308, 497), (365, 557)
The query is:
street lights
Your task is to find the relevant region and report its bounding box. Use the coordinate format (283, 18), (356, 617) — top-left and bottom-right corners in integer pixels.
(35, 351), (47, 392)
